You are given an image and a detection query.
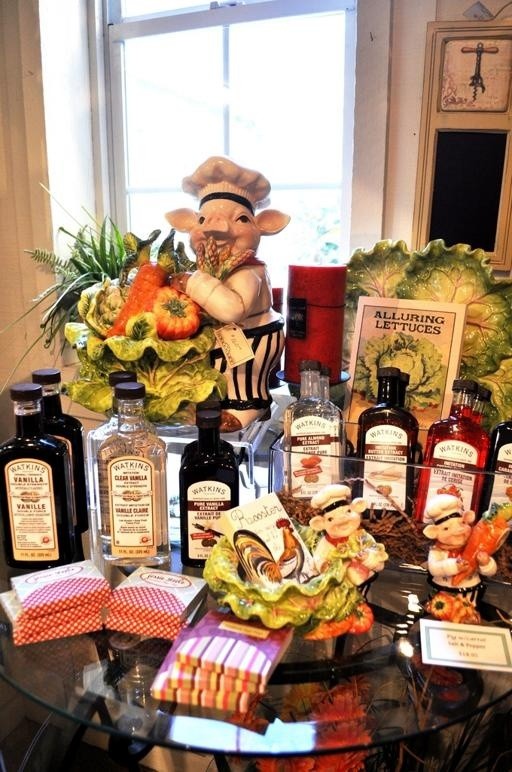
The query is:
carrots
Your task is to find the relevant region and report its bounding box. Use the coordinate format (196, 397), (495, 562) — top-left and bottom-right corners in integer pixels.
(107, 263), (167, 340)
(451, 516), (511, 589)
(303, 615), (352, 641)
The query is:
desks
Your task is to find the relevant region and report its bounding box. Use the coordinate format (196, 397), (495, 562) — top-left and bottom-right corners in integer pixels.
(0, 546), (512, 757)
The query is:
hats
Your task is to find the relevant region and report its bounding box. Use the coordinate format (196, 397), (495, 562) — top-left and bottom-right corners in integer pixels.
(424, 492), (463, 526)
(180, 154), (272, 216)
(311, 482), (353, 516)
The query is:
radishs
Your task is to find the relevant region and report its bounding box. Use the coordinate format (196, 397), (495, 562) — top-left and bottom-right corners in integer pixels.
(346, 555), (369, 588)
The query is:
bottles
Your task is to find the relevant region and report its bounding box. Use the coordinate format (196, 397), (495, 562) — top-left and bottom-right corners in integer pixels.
(317, 365), (346, 484)
(97, 382), (172, 567)
(350, 372), (419, 501)
(89, 371), (159, 545)
(1, 383), (77, 569)
(412, 378), (483, 525)
(182, 400), (236, 460)
(180, 411), (239, 569)
(358, 367), (415, 526)
(478, 421), (511, 543)
(282, 358), (337, 528)
(30, 367), (88, 534)
(470, 386), (492, 488)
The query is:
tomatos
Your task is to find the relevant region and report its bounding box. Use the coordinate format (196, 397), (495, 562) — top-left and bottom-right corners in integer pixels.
(349, 604), (374, 635)
(153, 287), (202, 341)
(431, 595), (454, 621)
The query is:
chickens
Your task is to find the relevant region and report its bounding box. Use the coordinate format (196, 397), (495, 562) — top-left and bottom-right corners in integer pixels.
(231, 518), (308, 586)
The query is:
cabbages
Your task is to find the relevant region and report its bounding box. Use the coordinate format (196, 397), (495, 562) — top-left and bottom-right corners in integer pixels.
(352, 331), (447, 412)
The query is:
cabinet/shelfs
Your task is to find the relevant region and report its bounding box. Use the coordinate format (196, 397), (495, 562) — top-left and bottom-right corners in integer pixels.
(410, 20), (512, 271)
